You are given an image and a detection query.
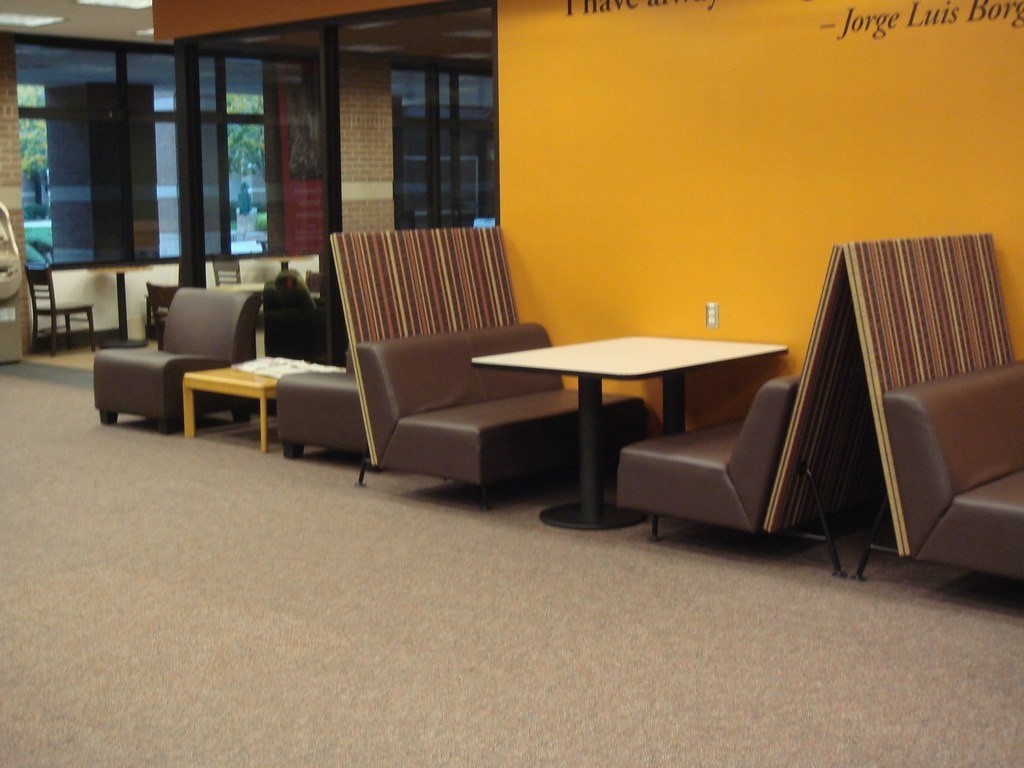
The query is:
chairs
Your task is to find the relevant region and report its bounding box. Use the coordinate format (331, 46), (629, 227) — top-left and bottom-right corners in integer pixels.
(24, 265), (95, 358)
(146, 282), (177, 351)
(213, 255), (241, 285)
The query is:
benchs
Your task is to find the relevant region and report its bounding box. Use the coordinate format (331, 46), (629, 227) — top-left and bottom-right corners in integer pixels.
(858, 361), (1024, 595)
(619, 374), (884, 577)
(276, 326), (645, 513)
(93, 286), (263, 435)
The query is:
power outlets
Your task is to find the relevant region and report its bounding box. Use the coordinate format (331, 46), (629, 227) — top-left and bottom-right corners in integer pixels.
(707, 303), (718, 328)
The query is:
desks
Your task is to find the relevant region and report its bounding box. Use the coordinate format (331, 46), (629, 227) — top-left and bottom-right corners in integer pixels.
(183, 362), (347, 453)
(91, 265), (153, 349)
(470, 335), (788, 531)
